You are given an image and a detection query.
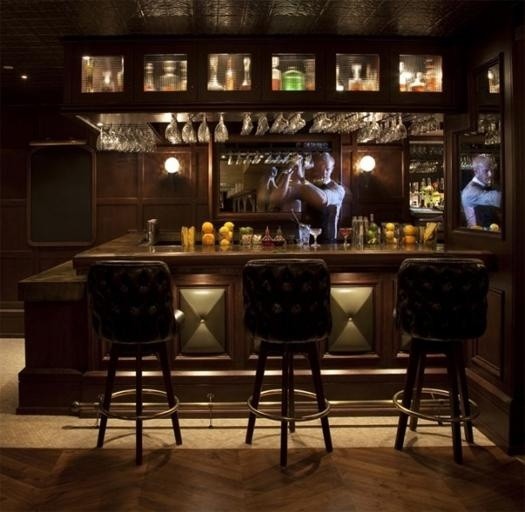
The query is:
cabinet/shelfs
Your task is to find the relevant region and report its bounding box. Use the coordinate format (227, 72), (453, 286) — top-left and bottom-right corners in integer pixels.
(57, 40), (456, 117)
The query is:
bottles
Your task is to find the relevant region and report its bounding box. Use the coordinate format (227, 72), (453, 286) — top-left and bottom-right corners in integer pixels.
(83, 57), (124, 94)
(272, 57), (315, 91)
(410, 176), (444, 207)
(208, 55), (251, 91)
(262, 225), (284, 245)
(400, 58), (437, 92)
(144, 59), (187, 92)
(335, 60), (380, 91)
(351, 213), (378, 250)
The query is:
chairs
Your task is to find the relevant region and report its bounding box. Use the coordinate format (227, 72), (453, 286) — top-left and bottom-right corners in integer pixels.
(87, 252), (186, 467)
(388, 250), (490, 465)
(240, 255), (335, 468)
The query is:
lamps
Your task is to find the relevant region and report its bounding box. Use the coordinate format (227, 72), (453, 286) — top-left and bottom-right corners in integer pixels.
(165, 156), (182, 185)
(359, 156), (376, 187)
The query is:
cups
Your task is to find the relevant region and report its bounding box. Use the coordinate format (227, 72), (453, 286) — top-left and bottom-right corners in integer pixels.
(239, 230), (261, 248)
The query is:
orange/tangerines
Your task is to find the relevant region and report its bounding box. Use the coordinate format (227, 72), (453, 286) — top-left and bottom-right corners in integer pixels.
(366, 229), (375, 240)
(370, 222), (378, 231)
(239, 226), (253, 234)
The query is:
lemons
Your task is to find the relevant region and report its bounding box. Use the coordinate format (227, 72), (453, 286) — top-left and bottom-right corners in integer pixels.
(489, 223), (499, 231)
(219, 221), (235, 246)
(385, 231), (394, 239)
(385, 223), (395, 231)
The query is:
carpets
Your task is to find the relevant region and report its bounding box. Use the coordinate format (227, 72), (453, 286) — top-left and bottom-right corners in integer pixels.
(0, 338), (496, 450)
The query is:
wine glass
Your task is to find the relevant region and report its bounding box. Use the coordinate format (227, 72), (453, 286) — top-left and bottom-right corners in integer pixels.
(94, 111), (444, 153)
(309, 227), (322, 248)
(339, 226), (352, 248)
(408, 145), (473, 174)
(475, 114), (502, 146)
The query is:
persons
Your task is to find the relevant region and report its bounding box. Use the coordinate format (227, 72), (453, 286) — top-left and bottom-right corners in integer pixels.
(255, 166), (302, 212)
(459, 152), (502, 229)
(270, 152), (346, 246)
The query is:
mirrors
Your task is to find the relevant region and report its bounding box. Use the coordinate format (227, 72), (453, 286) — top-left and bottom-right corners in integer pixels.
(212, 139), (343, 226)
(455, 106), (505, 239)
(24, 136), (99, 250)
(403, 136), (445, 223)
